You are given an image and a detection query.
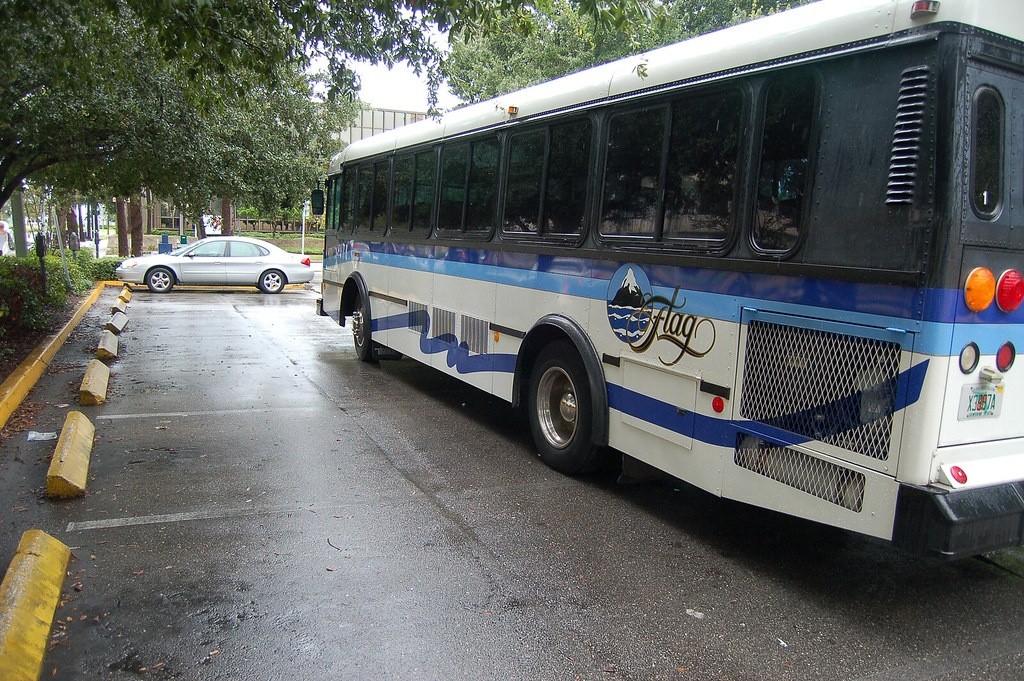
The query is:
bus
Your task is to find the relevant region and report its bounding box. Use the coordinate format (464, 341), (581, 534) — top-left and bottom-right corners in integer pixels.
(310, 0), (1024, 567)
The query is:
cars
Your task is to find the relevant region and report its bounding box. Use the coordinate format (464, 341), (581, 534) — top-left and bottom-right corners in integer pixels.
(115, 236), (315, 294)
